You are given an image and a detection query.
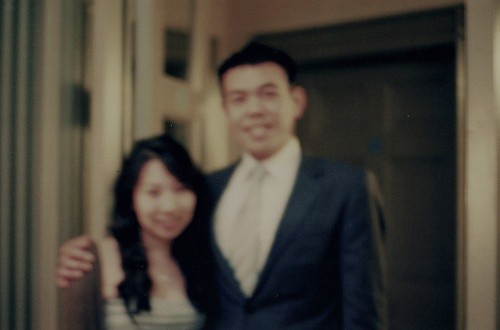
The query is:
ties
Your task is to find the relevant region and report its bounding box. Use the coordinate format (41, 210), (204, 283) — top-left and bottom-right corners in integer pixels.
(229, 164), (274, 290)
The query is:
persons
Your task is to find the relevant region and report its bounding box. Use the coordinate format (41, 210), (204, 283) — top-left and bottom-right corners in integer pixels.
(59, 38), (395, 330)
(58, 133), (251, 330)
